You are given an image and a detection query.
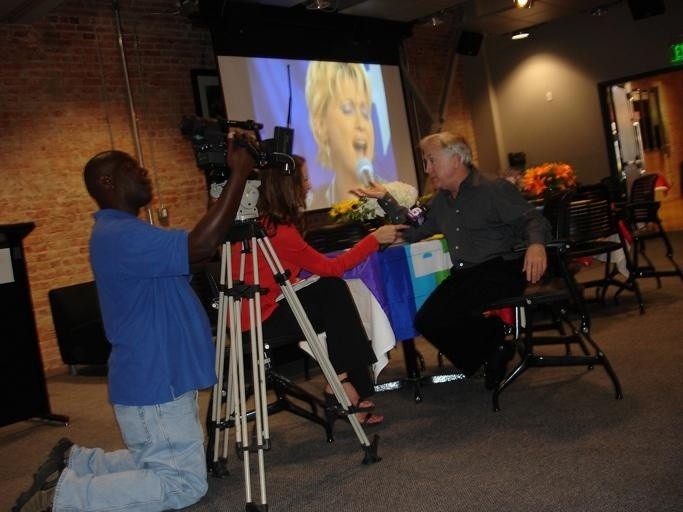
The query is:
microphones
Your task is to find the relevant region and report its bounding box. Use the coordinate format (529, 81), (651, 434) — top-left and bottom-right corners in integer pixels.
(355, 158), (375, 189)
(380, 204), (430, 253)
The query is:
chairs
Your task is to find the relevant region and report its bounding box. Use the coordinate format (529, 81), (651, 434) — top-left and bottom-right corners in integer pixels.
(471, 243), (623, 411)
(193, 272), (356, 467)
(524, 172), (682, 315)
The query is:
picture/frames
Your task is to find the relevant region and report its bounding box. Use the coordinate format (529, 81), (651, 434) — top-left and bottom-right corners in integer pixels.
(189, 69), (228, 122)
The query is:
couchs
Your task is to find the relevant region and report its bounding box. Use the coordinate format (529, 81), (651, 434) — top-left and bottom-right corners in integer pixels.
(45, 219), (388, 377)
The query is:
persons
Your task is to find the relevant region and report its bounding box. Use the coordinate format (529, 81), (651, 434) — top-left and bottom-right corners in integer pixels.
(300, 58), (417, 211)
(9, 130), (260, 511)
(345, 131), (555, 392)
(216, 150), (411, 430)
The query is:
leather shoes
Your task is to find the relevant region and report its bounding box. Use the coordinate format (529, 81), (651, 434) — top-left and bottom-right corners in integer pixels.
(482, 341), (523, 390)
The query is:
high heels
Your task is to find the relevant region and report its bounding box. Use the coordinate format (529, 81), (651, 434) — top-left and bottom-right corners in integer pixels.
(323, 378), (384, 427)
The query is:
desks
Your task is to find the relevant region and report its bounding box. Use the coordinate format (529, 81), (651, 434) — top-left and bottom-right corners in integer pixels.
(298, 232), (458, 404)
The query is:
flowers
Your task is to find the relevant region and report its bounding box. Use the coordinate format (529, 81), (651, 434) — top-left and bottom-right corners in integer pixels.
(330, 180), (417, 230)
(522, 161), (576, 198)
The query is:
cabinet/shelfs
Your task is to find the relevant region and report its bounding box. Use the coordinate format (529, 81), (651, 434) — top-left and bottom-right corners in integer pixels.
(0, 222), (70, 430)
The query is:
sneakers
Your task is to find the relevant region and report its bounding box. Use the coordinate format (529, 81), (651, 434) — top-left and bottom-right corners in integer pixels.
(12, 438), (75, 511)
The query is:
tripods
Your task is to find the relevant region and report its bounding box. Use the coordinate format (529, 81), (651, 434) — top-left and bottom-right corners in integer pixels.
(212, 218), (381, 512)
